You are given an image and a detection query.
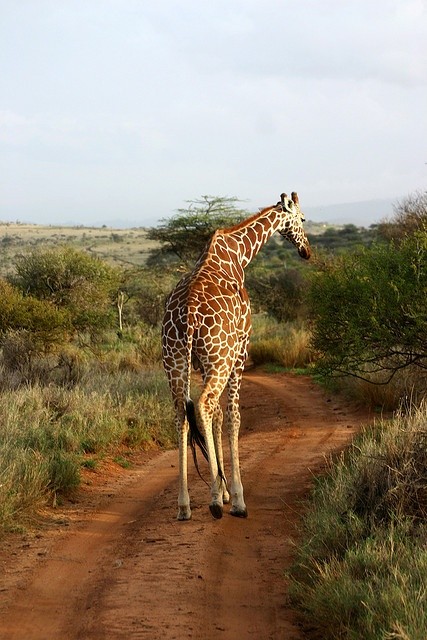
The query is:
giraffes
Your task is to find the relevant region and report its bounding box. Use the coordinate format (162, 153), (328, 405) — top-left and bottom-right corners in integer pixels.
(161, 191), (311, 521)
